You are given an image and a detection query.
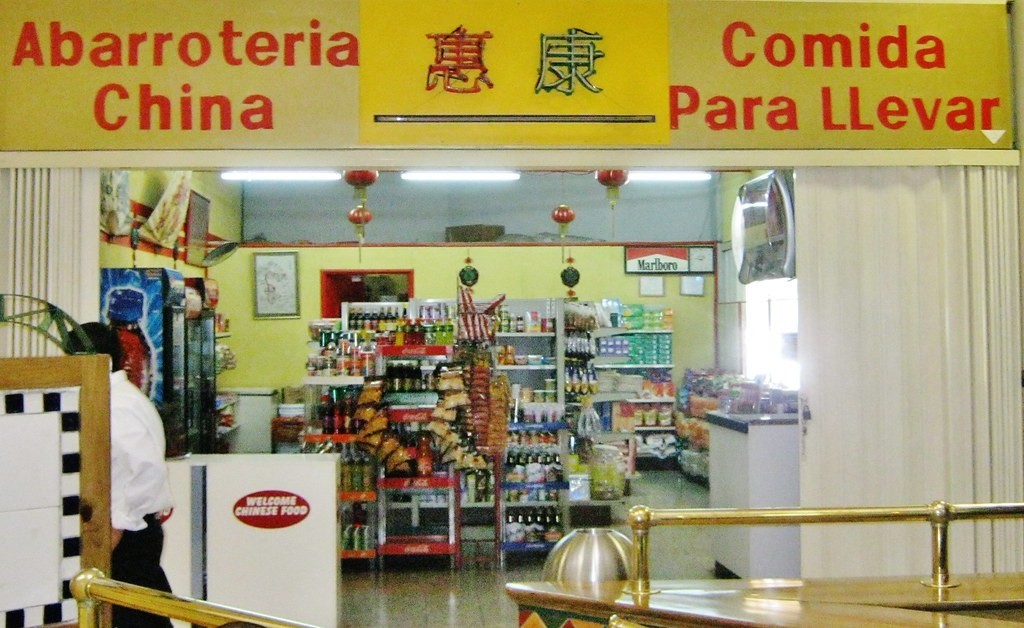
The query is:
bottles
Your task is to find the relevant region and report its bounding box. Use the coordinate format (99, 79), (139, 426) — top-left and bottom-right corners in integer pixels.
(675, 392), (741, 478)
(279, 293), (623, 550)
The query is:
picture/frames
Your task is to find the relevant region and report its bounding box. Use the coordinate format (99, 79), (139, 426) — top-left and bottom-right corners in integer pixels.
(250, 251), (301, 319)
(186, 189), (210, 269)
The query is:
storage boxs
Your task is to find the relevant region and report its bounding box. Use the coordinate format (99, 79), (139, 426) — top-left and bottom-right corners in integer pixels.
(599, 334), (673, 366)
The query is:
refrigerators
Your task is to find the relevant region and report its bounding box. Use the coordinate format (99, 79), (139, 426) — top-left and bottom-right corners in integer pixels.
(706, 409), (802, 579)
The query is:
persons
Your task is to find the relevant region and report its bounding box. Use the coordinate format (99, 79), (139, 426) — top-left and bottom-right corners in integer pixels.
(65, 321), (173, 628)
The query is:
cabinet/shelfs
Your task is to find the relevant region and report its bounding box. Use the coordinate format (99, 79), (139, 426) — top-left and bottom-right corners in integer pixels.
(674, 403), (714, 485)
(590, 328), (680, 468)
(304, 298), (572, 567)
(378, 463), (460, 579)
